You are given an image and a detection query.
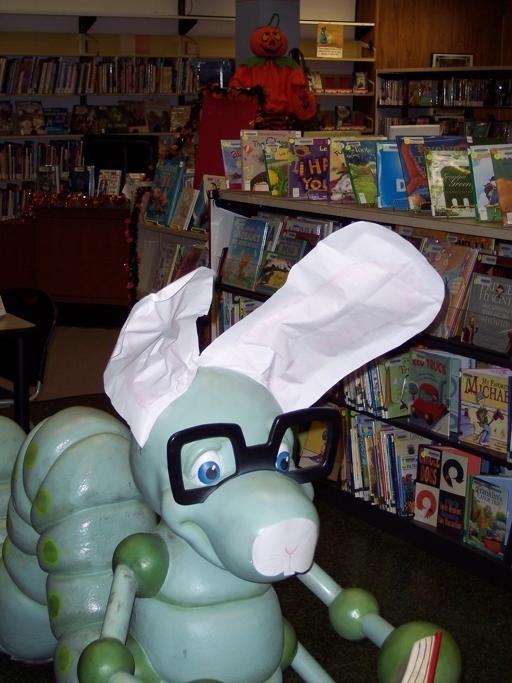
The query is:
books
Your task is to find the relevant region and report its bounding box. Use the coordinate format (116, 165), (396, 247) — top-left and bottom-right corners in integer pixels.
(316, 23), (345, 59)
(0, 56), (201, 221)
(218, 124), (512, 226)
(380, 80), (512, 140)
(308, 71), (367, 129)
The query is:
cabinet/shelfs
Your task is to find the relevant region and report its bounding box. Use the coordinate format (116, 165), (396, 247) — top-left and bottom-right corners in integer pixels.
(1, 49), (512, 571)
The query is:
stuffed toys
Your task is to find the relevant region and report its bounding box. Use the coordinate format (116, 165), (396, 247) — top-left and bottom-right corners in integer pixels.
(228, 10), (317, 121)
